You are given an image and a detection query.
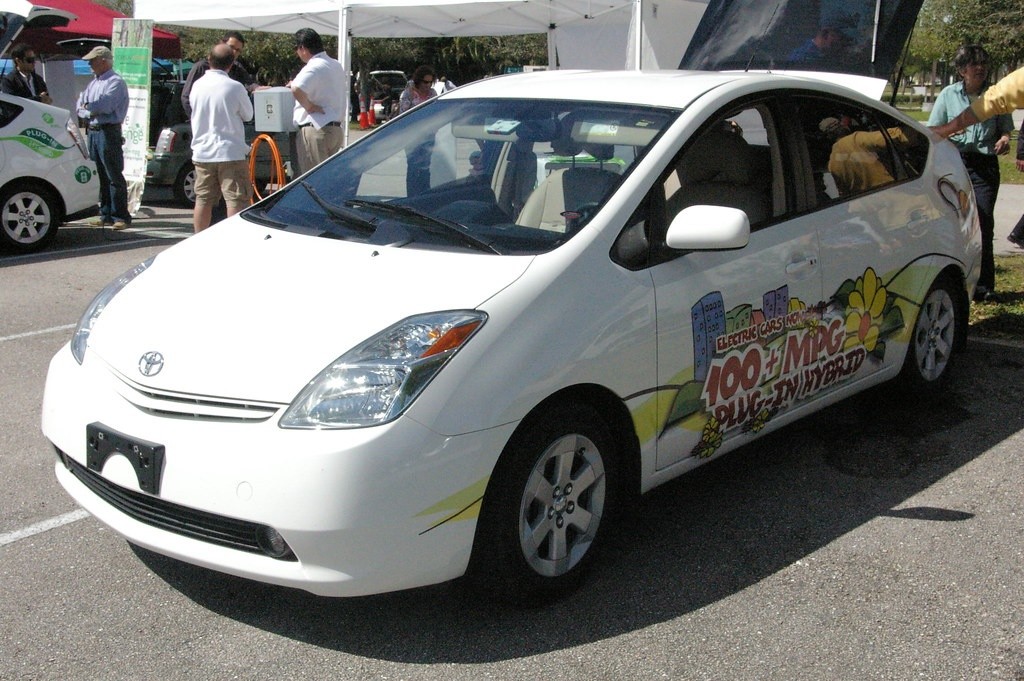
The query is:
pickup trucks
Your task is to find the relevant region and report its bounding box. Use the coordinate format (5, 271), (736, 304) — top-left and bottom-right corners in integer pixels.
(359, 70), (414, 124)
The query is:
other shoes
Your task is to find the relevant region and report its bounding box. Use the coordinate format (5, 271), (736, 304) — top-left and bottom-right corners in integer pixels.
(112, 221), (130, 230)
(89, 218), (115, 227)
(982, 289), (1000, 303)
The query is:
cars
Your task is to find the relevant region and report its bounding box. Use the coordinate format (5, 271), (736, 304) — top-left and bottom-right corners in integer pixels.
(136, 78), (298, 207)
(38, 2), (986, 609)
(0, 1), (103, 256)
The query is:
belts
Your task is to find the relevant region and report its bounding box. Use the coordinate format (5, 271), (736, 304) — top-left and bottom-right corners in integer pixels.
(89, 124), (115, 131)
(299, 121), (341, 126)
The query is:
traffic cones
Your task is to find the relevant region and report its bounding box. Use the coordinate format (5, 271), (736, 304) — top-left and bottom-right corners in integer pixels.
(360, 98), (369, 130)
(368, 95), (377, 126)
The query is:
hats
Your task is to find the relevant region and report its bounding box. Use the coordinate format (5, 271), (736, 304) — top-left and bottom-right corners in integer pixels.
(81, 46), (113, 61)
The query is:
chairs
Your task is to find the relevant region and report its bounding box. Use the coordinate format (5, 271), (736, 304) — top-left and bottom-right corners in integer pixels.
(517, 112), (626, 234)
(657, 127), (778, 244)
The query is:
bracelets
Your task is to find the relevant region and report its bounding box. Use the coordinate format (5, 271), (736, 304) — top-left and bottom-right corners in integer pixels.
(84, 102), (89, 109)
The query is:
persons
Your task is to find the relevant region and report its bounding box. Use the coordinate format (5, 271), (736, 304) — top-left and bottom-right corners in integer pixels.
(76, 46), (131, 230)
(819, 119), (909, 196)
(929, 66), (1024, 138)
(469, 151), (483, 175)
(287, 28), (346, 175)
(928, 44), (1015, 301)
(0, 44), (52, 120)
(374, 76), (392, 115)
(351, 70), (360, 122)
(187, 45), (253, 233)
(399, 66), (437, 195)
(181, 32), (272, 217)
(786, 27), (842, 73)
(836, 188), (945, 262)
(441, 77), (457, 90)
(1008, 119), (1024, 248)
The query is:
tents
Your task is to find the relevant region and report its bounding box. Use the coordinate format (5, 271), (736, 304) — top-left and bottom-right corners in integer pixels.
(173, 60), (192, 80)
(132, 0), (709, 136)
(30, 0), (180, 61)
(0, 59), (174, 75)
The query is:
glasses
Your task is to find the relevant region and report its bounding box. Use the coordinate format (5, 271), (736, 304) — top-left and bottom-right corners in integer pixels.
(230, 45), (242, 52)
(294, 45), (302, 51)
(423, 81), (433, 84)
(22, 57), (36, 63)
